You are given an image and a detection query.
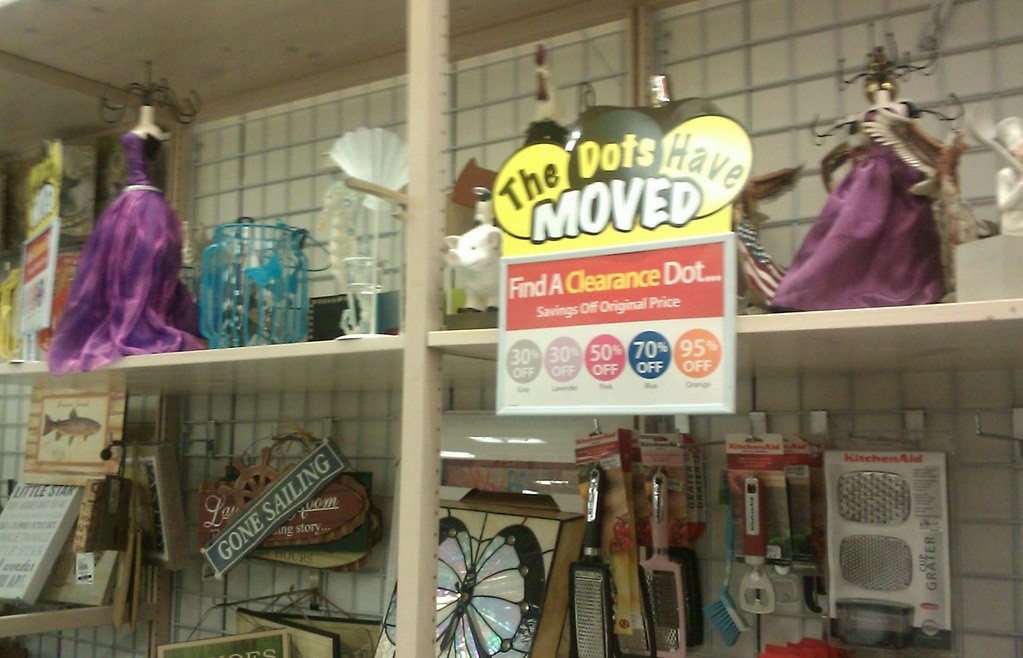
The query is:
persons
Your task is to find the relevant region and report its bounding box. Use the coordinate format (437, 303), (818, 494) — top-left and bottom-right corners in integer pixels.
(47, 106), (209, 372)
(769, 90), (947, 313)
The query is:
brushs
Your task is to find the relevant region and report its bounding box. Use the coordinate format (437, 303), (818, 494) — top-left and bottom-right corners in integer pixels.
(702, 503), (751, 647)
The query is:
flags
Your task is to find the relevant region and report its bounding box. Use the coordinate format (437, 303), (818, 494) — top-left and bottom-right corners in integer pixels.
(736, 210), (789, 305)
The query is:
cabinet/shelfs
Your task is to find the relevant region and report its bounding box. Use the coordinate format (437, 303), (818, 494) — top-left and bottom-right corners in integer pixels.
(1, 1), (1022, 658)
